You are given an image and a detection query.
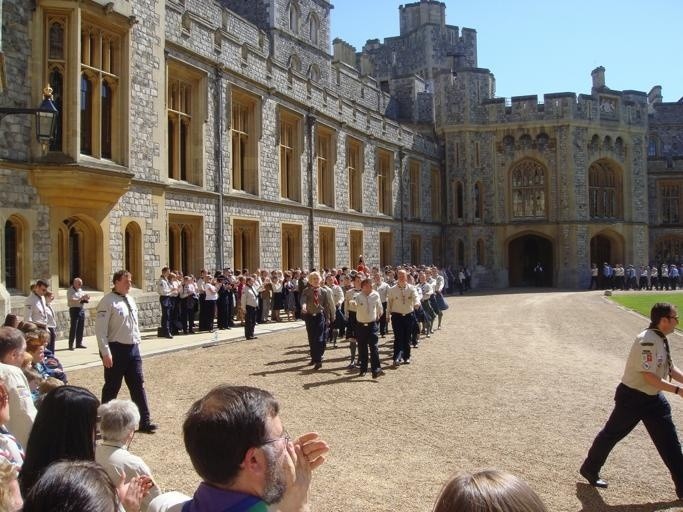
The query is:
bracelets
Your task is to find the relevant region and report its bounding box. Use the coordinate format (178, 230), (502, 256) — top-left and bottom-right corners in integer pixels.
(674, 386), (680, 394)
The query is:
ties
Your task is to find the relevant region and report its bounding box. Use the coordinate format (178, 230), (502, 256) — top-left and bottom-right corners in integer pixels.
(314, 288), (319, 308)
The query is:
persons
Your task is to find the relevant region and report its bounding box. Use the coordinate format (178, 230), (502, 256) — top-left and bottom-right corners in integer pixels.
(300, 263), (449, 378)
(578, 303), (683, 498)
(23, 277), (57, 355)
(176, 383), (332, 511)
(158, 267), (321, 339)
(432, 469), (546, 511)
(66, 278), (89, 350)
(533, 261), (683, 291)
(446, 265), (473, 295)
(0, 314), (190, 511)
(93, 270), (160, 430)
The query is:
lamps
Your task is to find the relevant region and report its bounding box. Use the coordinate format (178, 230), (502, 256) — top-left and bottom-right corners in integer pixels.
(0, 81), (58, 144)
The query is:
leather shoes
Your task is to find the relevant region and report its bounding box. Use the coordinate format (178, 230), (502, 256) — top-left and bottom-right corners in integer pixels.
(308, 325), (434, 379)
(580, 464), (608, 487)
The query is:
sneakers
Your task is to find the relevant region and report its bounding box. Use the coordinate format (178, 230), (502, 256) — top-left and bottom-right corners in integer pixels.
(140, 423), (157, 430)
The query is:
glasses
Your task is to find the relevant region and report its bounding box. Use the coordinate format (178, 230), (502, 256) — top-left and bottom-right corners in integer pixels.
(258, 431), (290, 447)
